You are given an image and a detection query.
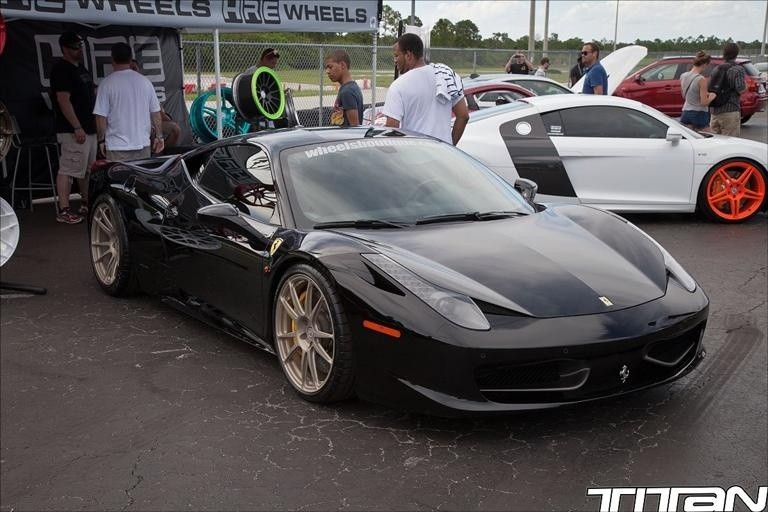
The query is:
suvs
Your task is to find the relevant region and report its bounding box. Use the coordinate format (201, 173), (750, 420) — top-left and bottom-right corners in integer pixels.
(610, 54), (767, 126)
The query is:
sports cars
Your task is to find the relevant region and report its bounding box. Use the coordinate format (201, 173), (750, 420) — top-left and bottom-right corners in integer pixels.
(78, 125), (712, 428)
(374, 88), (766, 227)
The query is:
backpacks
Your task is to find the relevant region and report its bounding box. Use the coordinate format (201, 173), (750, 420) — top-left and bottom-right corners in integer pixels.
(706, 62), (735, 109)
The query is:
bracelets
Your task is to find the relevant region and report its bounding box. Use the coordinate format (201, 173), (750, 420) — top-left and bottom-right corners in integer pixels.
(156, 134), (165, 142)
(73, 126), (83, 131)
(98, 139), (107, 146)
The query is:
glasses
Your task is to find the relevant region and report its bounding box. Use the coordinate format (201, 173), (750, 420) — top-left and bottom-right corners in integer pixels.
(66, 43), (84, 50)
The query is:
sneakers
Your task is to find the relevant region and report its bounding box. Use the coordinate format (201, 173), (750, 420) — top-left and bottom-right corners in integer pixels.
(55, 207), (85, 225)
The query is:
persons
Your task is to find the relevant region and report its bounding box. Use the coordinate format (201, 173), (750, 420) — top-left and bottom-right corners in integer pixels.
(537, 56), (551, 79)
(48, 30), (97, 226)
(245, 47), (292, 127)
(91, 40), (164, 163)
(677, 49), (716, 125)
(708, 42), (746, 135)
(580, 43), (609, 96)
(323, 49), (364, 126)
(381, 32), (470, 147)
(504, 51), (533, 75)
(569, 50), (586, 86)
(129, 59), (183, 147)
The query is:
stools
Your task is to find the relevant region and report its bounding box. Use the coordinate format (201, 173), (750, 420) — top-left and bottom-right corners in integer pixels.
(11, 135), (58, 216)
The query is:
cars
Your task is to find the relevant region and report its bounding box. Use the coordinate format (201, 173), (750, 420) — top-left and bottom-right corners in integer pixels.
(361, 80), (538, 128)
(460, 43), (657, 135)
(753, 62), (767, 90)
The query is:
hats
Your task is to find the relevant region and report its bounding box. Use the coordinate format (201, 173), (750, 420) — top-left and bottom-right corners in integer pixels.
(58, 32), (86, 46)
